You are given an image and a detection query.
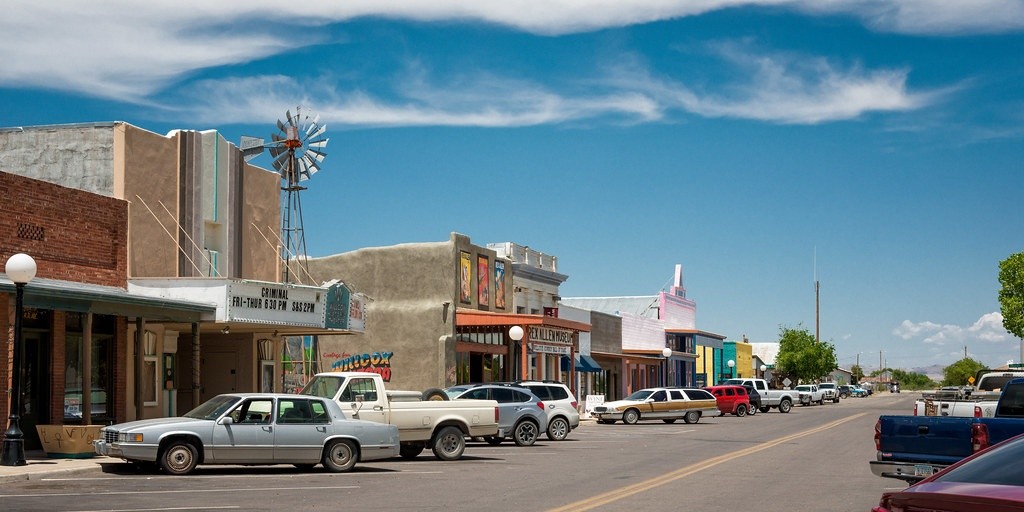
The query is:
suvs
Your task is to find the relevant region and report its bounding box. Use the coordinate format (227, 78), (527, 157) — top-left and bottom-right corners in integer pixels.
(441, 380), (580, 447)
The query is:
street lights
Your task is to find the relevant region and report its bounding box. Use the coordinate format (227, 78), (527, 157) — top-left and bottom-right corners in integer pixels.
(760, 364), (767, 379)
(509, 325), (525, 381)
(0, 252), (37, 467)
(663, 347), (672, 387)
(880, 350), (888, 393)
(727, 360), (735, 379)
(857, 352), (863, 385)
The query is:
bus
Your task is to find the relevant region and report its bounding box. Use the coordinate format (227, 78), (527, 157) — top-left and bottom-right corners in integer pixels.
(889, 382), (901, 393)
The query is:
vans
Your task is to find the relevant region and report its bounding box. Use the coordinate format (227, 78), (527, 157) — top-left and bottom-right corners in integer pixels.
(590, 387), (722, 424)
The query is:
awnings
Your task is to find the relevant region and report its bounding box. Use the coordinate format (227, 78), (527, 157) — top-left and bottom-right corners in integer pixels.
(561, 355), (602, 372)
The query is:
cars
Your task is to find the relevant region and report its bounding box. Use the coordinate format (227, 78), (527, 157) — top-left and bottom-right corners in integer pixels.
(698, 385), (761, 417)
(64, 413), (81, 419)
(837, 385), (874, 399)
(869, 367), (1024, 512)
(818, 383), (841, 403)
(93, 392), (400, 476)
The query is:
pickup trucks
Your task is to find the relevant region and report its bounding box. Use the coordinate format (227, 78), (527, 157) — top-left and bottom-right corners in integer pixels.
(719, 378), (801, 413)
(264, 372), (499, 460)
(794, 384), (827, 406)
